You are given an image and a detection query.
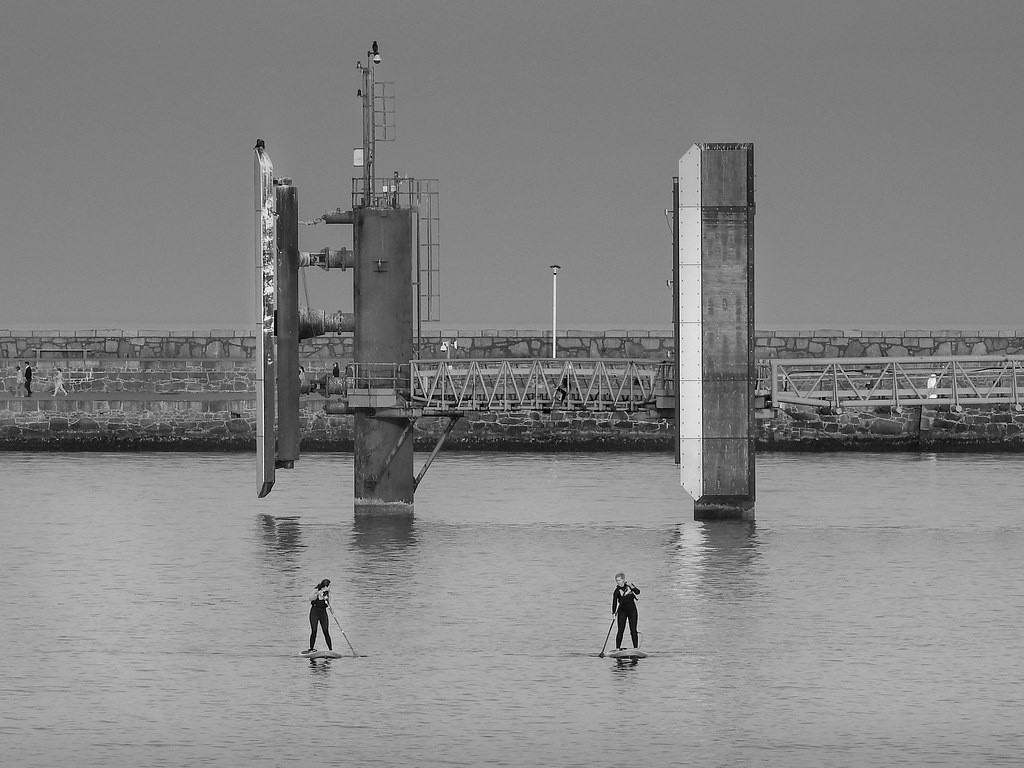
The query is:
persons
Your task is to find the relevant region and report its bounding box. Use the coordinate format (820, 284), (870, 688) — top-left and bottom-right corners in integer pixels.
(333, 362), (339, 383)
(14, 366), (24, 397)
(52, 368), (67, 396)
(611, 573), (641, 650)
(309, 579), (332, 650)
(24, 361), (33, 397)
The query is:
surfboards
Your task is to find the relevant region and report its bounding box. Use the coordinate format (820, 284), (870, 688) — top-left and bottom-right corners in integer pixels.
(298, 648), (341, 659)
(607, 647), (648, 659)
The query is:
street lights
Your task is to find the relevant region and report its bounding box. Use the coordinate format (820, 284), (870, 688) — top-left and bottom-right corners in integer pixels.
(550, 265), (561, 359)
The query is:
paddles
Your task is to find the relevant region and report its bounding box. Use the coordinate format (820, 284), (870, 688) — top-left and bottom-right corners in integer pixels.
(598, 586), (628, 659)
(327, 600), (357, 656)
